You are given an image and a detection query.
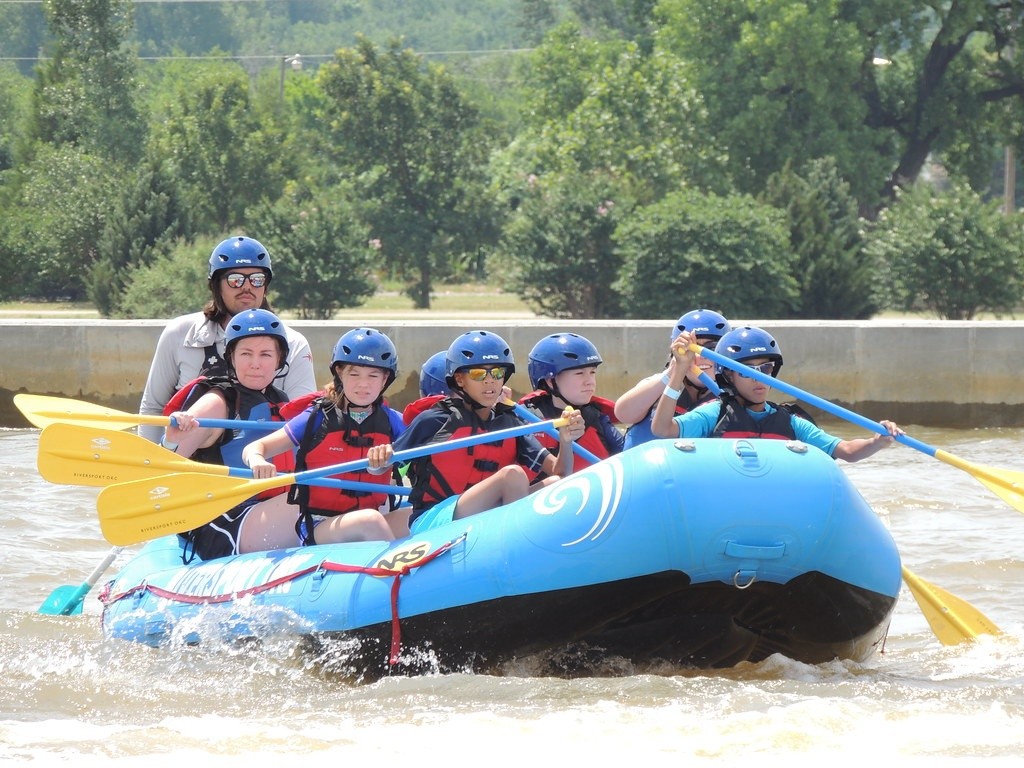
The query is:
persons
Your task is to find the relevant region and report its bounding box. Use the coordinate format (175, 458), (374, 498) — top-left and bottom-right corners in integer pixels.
(368, 329), (586, 537)
(614, 307), (732, 454)
(169, 308), (304, 561)
(242, 327), (414, 541)
(137, 236), (317, 449)
(513, 332), (626, 485)
(651, 324), (907, 463)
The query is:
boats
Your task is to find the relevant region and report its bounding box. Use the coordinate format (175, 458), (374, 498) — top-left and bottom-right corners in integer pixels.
(98, 437), (904, 671)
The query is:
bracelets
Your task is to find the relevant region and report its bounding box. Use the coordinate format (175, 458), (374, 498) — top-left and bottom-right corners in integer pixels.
(246, 450), (264, 468)
(160, 434), (180, 454)
(663, 385), (685, 400)
(162, 439), (178, 451)
(660, 371), (671, 386)
(369, 466), (380, 471)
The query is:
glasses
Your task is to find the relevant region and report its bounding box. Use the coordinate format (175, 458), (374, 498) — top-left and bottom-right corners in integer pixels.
(221, 272), (269, 288)
(738, 361), (776, 378)
(460, 366), (508, 381)
(704, 341), (717, 352)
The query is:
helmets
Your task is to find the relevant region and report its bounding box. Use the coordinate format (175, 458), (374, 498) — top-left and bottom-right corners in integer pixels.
(329, 327), (398, 372)
(670, 308), (732, 341)
(714, 326), (783, 374)
(223, 308), (289, 359)
(208, 236), (272, 280)
(528, 332), (603, 392)
(419, 351), (454, 398)
(445, 330), (515, 391)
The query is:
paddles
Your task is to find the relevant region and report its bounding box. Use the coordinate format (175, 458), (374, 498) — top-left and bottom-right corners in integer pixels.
(96, 402), (574, 547)
(499, 396), (602, 465)
(688, 362), (1005, 648)
(34, 422), (412, 497)
(677, 337), (1024, 512)
(38, 548), (125, 616)
(12, 392), (290, 433)
